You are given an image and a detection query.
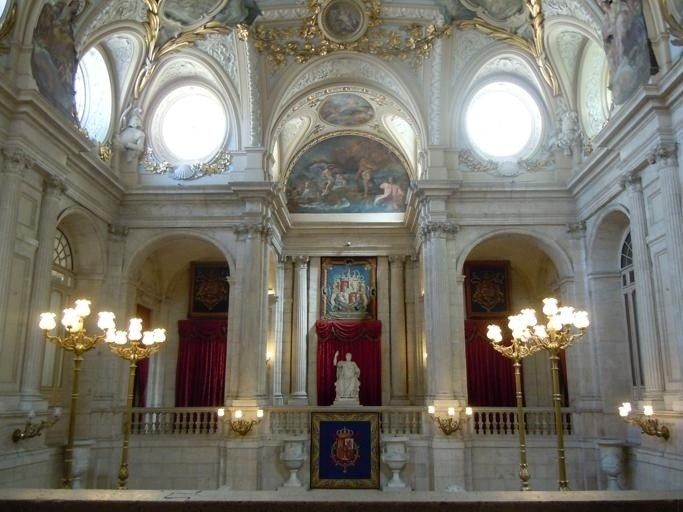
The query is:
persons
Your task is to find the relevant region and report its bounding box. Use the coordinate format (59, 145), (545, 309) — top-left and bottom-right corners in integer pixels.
(354, 159), (376, 198)
(319, 162), (337, 197)
(374, 175), (404, 209)
(332, 351), (362, 399)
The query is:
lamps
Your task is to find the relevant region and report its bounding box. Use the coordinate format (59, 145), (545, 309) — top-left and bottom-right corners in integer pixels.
(216, 405), (263, 438)
(10, 406), (64, 444)
(617, 401), (671, 442)
(427, 404), (473, 435)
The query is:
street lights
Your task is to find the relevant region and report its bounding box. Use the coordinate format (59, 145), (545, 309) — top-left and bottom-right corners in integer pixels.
(35, 297), (114, 488)
(519, 297), (589, 491)
(485, 314), (550, 492)
(108, 316), (166, 488)
